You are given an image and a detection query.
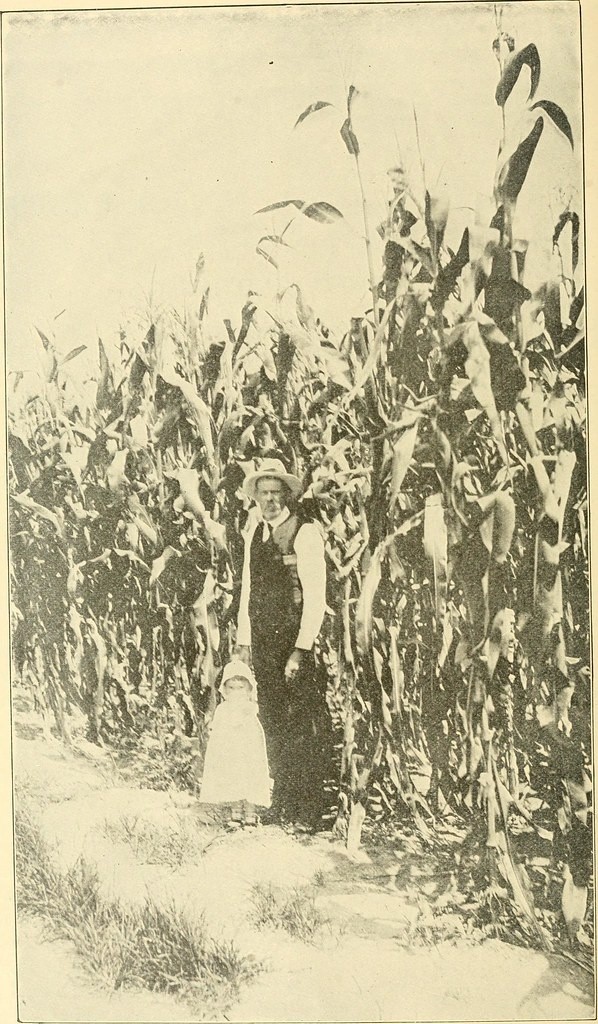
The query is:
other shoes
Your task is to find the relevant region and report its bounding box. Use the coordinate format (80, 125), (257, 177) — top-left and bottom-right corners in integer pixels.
(224, 817), (257, 834)
(288, 821), (329, 835)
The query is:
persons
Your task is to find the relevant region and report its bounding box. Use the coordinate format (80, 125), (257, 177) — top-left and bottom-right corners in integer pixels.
(236, 459), (345, 835)
(201, 660), (275, 830)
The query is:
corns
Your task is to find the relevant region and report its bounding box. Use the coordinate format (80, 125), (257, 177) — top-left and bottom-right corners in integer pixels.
(118, 479), (205, 554)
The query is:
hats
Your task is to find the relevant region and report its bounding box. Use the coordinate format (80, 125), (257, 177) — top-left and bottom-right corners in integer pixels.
(243, 458), (302, 501)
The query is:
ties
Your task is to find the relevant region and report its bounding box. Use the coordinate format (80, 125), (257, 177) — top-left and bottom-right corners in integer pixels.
(267, 523), (274, 538)
